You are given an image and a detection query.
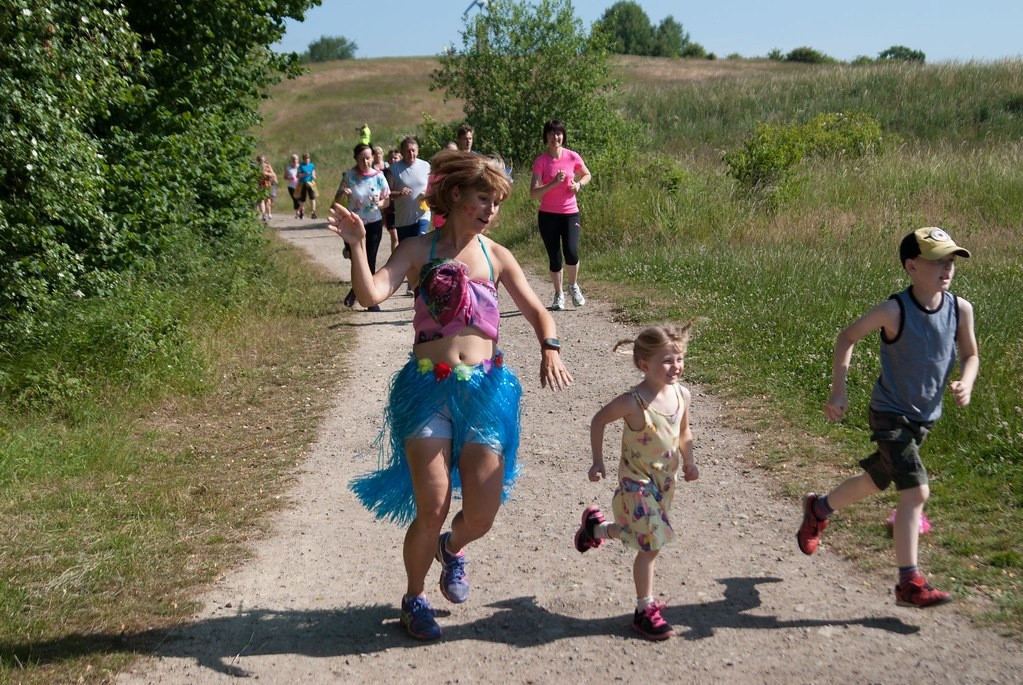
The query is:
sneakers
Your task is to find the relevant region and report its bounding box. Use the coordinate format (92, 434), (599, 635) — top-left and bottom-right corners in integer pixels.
(632, 598), (674, 640)
(567, 284), (585, 306)
(399, 591), (442, 641)
(551, 292), (564, 310)
(798, 492), (830, 555)
(435, 531), (470, 604)
(573, 504), (607, 553)
(894, 577), (952, 608)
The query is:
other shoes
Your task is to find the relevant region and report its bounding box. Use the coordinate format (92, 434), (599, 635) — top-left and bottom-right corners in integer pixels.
(344, 288), (355, 307)
(294, 213), (300, 219)
(407, 285), (414, 295)
(262, 214), (266, 220)
(367, 305), (380, 312)
(311, 213), (317, 219)
(268, 214), (273, 220)
(299, 212), (304, 219)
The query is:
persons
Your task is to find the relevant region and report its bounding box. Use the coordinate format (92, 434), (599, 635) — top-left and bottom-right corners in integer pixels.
(254, 124), (473, 312)
(575, 318), (700, 641)
(328, 149), (574, 641)
(530, 119), (591, 311)
(796, 226), (980, 609)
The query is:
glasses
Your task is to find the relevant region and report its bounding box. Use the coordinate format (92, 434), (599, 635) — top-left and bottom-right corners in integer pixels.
(920, 256), (956, 268)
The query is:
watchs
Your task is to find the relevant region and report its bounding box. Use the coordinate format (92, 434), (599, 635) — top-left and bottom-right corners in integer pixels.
(578, 181), (585, 190)
(541, 338), (560, 353)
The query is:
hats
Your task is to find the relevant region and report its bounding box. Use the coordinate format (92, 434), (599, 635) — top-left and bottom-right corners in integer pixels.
(898, 226), (970, 260)
